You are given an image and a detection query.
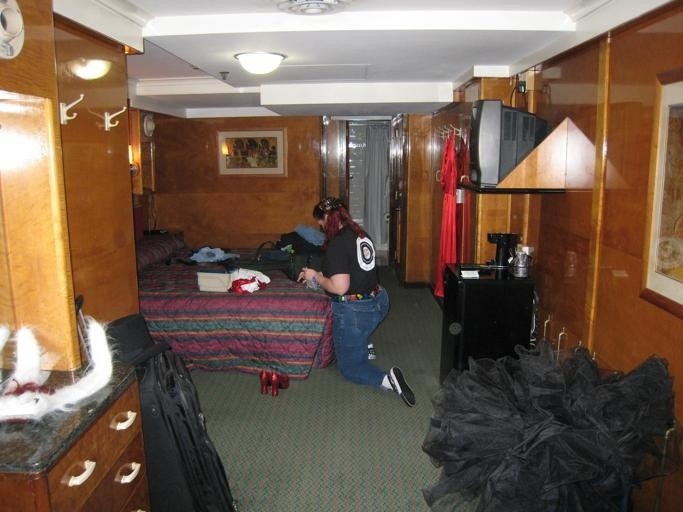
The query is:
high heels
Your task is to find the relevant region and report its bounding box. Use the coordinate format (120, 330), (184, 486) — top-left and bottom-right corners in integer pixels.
(261, 370), (289, 396)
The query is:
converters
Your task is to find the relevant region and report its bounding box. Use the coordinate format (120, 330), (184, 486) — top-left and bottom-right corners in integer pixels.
(518, 81), (526, 93)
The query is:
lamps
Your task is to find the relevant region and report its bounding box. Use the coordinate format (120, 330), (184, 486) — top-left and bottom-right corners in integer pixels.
(64, 56), (117, 80)
(234, 50), (288, 75)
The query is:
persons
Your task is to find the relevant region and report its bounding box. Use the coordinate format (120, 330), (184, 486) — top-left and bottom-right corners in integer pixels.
(298, 197), (416, 407)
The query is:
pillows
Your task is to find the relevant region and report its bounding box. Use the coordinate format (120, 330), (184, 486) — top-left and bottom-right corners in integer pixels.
(135, 233), (186, 273)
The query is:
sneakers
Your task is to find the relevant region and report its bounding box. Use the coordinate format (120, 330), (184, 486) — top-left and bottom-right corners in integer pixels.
(368, 343), (376, 360)
(389, 366), (415, 406)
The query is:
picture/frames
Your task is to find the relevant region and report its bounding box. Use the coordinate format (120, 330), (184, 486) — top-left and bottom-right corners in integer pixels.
(639, 68), (683, 321)
(216, 126), (288, 178)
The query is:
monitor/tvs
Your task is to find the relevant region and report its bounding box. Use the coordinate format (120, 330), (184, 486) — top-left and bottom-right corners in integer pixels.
(468, 98), (548, 192)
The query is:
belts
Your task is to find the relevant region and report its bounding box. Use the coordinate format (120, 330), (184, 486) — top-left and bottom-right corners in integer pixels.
(335, 286), (379, 302)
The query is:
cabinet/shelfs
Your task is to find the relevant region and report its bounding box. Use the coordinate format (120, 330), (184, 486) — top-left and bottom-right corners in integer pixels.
(439, 264), (536, 388)
(0, 372), (151, 512)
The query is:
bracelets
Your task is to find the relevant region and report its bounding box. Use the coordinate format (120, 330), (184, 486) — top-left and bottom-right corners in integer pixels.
(312, 275), (320, 284)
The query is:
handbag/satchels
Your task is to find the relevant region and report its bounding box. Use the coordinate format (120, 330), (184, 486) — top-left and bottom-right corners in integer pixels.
(289, 261), (321, 282)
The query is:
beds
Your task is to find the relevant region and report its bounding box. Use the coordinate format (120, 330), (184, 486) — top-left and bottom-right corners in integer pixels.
(136, 249), (336, 381)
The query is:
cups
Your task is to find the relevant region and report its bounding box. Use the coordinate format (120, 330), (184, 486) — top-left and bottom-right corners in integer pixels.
(512, 267), (529, 278)
(515, 252), (532, 267)
